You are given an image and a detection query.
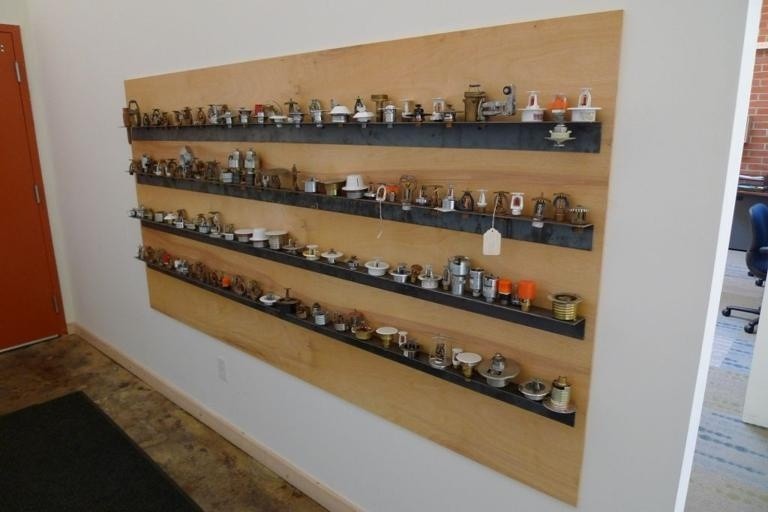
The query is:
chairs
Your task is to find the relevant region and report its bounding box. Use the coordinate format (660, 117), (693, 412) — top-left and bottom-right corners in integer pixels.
(721, 203), (768, 335)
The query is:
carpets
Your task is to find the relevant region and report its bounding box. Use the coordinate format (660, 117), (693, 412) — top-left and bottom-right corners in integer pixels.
(0, 387), (210, 510)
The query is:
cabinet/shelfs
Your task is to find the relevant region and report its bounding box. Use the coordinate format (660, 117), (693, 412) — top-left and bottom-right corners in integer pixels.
(126, 118), (601, 428)
